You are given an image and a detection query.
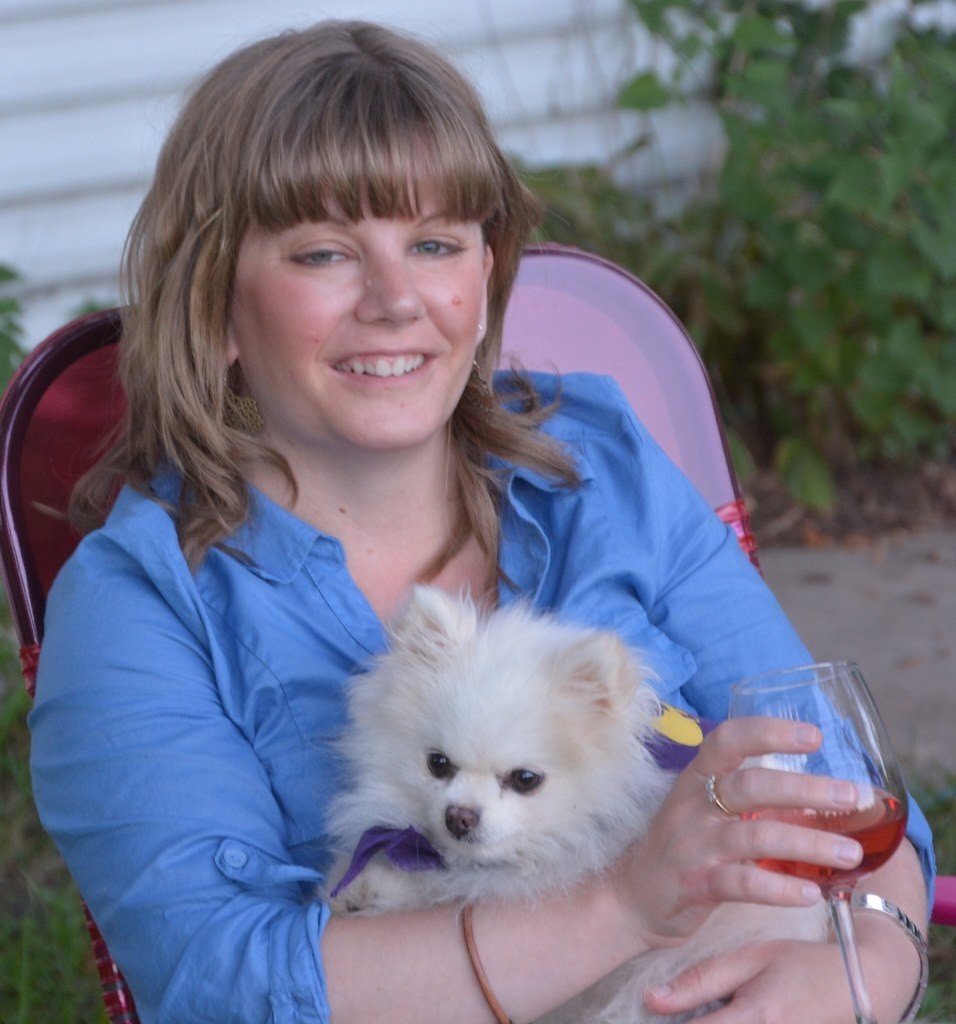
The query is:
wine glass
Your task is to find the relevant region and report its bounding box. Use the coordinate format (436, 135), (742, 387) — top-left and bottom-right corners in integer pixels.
(728, 658), (909, 1024)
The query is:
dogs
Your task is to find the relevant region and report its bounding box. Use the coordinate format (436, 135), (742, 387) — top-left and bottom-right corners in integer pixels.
(311, 586), (833, 1024)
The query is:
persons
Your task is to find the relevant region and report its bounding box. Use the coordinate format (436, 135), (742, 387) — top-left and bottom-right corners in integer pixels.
(29, 18), (936, 1023)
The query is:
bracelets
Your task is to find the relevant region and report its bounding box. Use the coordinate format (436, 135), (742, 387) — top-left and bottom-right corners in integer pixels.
(854, 893), (929, 1019)
(460, 902), (512, 1021)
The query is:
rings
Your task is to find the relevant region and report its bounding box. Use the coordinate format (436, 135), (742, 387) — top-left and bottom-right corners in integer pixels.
(702, 773), (726, 815)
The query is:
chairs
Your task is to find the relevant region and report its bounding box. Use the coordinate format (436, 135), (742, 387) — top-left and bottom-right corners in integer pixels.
(2, 240), (956, 1024)
